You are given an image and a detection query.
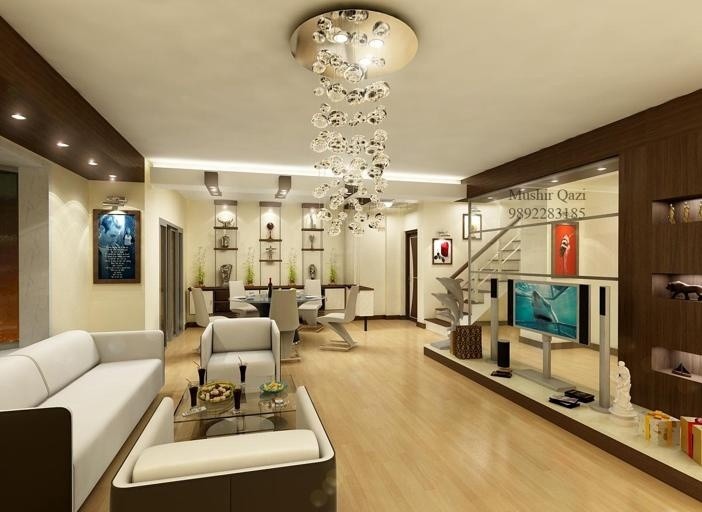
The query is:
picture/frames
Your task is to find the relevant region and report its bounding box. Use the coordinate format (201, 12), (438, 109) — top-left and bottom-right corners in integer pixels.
(91, 208), (141, 285)
(432, 238), (453, 265)
(463, 214), (482, 240)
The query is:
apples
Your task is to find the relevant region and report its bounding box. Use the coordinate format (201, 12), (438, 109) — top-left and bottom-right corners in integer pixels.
(441, 242), (449, 257)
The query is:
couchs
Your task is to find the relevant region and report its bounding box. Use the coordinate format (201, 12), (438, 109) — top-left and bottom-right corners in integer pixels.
(0, 329), (164, 512)
(198, 316), (282, 390)
(109, 383), (337, 511)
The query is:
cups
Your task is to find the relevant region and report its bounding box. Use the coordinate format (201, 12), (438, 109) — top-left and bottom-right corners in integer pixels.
(187, 363), (247, 414)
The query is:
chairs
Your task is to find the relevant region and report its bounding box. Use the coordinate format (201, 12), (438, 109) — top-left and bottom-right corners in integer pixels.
(190, 279), (359, 362)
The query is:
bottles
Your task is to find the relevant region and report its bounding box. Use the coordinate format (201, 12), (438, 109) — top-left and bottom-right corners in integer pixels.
(267, 278), (273, 298)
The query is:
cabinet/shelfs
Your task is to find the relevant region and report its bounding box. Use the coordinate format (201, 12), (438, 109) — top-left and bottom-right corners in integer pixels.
(259, 239), (282, 262)
(301, 229), (324, 251)
(651, 193), (701, 383)
(213, 227), (238, 250)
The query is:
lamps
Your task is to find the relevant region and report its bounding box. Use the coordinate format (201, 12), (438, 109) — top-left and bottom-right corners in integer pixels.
(285, 5), (421, 234)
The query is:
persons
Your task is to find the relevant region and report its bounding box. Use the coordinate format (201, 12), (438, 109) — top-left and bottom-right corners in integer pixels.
(98, 215), (123, 279)
(612, 361), (632, 406)
(123, 227), (135, 253)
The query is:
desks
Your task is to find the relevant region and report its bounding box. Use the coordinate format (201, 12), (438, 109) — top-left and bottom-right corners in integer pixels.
(172, 373), (298, 440)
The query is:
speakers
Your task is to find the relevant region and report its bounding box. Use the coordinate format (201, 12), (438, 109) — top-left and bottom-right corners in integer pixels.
(600, 286), (606, 316)
(579, 284), (592, 346)
(490, 278), (498, 298)
(498, 339), (510, 368)
(508, 279), (513, 326)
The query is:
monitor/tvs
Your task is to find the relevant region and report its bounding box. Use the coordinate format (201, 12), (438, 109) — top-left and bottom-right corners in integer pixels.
(513, 279), (579, 344)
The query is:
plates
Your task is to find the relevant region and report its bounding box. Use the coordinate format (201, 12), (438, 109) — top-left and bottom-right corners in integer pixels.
(305, 296), (317, 298)
(259, 382), (286, 394)
